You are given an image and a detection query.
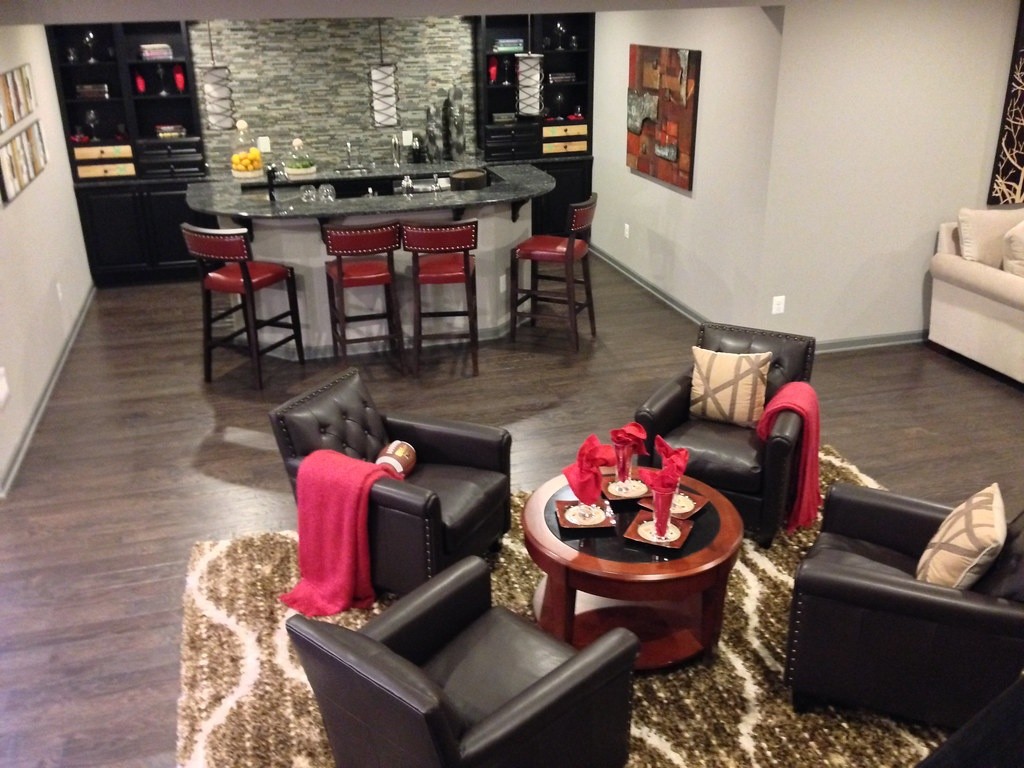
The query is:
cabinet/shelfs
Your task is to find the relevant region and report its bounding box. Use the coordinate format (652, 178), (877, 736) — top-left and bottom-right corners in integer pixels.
(43, 23), (228, 286)
(474, 13), (596, 247)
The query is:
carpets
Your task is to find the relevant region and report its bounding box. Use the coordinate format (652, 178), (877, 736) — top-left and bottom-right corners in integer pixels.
(174, 444), (959, 768)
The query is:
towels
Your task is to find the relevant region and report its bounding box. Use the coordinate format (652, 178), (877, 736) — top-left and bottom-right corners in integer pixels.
(561, 432), (618, 507)
(756, 381), (824, 536)
(611, 421), (650, 483)
(654, 435), (689, 495)
(276, 449), (404, 618)
(637, 457), (682, 537)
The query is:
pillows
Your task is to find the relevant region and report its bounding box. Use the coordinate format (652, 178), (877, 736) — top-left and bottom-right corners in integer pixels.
(957, 208), (1024, 275)
(917, 482), (1008, 589)
(690, 344), (773, 432)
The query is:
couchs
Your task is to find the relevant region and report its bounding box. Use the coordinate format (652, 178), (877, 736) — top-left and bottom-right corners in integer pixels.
(786, 484), (1024, 714)
(928, 222), (1024, 384)
(269, 366), (512, 593)
(634, 321), (815, 551)
(284, 555), (635, 768)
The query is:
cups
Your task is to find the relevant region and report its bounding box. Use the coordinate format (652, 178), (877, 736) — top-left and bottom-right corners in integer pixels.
(300, 184), (316, 202)
(614, 443), (632, 491)
(318, 184), (335, 202)
(544, 37), (550, 48)
(661, 456), (680, 508)
(577, 499), (593, 519)
(67, 47), (77, 61)
(652, 489), (674, 538)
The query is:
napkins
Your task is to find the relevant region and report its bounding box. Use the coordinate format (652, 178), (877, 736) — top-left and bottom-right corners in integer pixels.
(562, 421), (689, 537)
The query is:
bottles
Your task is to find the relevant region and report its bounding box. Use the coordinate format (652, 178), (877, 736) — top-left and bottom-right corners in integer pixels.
(392, 134), (400, 167)
(401, 176), (413, 195)
(569, 35), (577, 49)
(412, 137), (419, 163)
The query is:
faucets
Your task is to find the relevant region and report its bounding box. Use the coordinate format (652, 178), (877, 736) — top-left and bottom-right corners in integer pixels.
(367, 187), (373, 197)
(346, 142), (351, 168)
(391, 134), (400, 168)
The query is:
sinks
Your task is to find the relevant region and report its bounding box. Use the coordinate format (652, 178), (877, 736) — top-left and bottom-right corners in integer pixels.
(333, 168), (374, 176)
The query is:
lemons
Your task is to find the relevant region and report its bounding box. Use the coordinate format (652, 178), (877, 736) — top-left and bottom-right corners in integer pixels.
(231, 147), (262, 171)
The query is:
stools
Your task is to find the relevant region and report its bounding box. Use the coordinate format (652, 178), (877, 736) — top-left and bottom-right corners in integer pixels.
(320, 218), (411, 378)
(509, 193), (598, 351)
(398, 217), (481, 376)
(181, 223), (306, 393)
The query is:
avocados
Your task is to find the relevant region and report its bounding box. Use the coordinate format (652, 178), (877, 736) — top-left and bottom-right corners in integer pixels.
(287, 160), (316, 168)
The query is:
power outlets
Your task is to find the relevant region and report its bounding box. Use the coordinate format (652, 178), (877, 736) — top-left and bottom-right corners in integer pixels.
(624, 224), (629, 239)
(772, 296), (786, 314)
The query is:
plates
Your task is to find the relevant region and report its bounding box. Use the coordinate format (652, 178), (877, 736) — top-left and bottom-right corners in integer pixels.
(636, 488), (708, 519)
(623, 509), (694, 548)
(601, 477), (654, 500)
(555, 500), (617, 527)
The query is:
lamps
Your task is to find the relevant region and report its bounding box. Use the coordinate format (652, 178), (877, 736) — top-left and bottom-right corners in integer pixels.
(199, 22), (238, 132)
(367, 17), (400, 129)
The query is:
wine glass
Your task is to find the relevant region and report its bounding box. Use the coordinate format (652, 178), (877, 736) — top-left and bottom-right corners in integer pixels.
(552, 18), (566, 50)
(501, 57), (512, 85)
(81, 29), (97, 63)
(556, 91), (564, 120)
(155, 64), (169, 96)
(85, 107), (99, 141)
